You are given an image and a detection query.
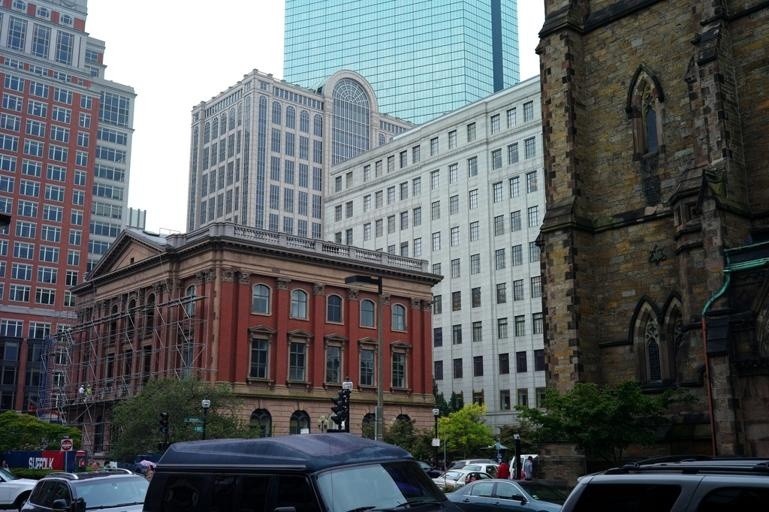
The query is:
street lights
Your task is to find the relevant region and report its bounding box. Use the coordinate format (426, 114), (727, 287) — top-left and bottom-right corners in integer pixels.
(0, 213), (12, 227)
(433, 408), (440, 468)
(317, 414), (328, 434)
(201, 396), (211, 440)
(511, 433), (522, 479)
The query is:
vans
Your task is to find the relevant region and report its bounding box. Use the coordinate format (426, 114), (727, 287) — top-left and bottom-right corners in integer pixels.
(143, 432), (472, 512)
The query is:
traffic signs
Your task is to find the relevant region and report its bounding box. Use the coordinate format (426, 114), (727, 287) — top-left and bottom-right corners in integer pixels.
(193, 426), (203, 433)
(184, 417), (204, 423)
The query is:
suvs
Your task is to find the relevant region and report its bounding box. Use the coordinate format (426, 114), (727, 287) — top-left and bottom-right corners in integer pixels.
(560, 454), (769, 512)
(20, 468), (150, 512)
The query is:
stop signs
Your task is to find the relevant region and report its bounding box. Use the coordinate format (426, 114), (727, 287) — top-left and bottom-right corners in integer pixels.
(60, 438), (73, 452)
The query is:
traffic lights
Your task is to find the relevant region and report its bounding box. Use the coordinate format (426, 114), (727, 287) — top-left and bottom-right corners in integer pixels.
(337, 388), (350, 424)
(159, 412), (169, 444)
(329, 396), (342, 426)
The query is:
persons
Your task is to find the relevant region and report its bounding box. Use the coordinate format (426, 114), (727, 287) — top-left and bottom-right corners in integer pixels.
(103, 460), (111, 472)
(78, 384), (85, 401)
(145, 464), (154, 481)
(496, 455), (534, 481)
(0, 458), (11, 473)
(85, 384), (92, 401)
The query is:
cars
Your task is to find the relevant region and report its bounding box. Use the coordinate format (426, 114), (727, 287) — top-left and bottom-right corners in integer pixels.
(414, 459), (501, 494)
(443, 478), (568, 512)
(0, 468), (39, 512)
